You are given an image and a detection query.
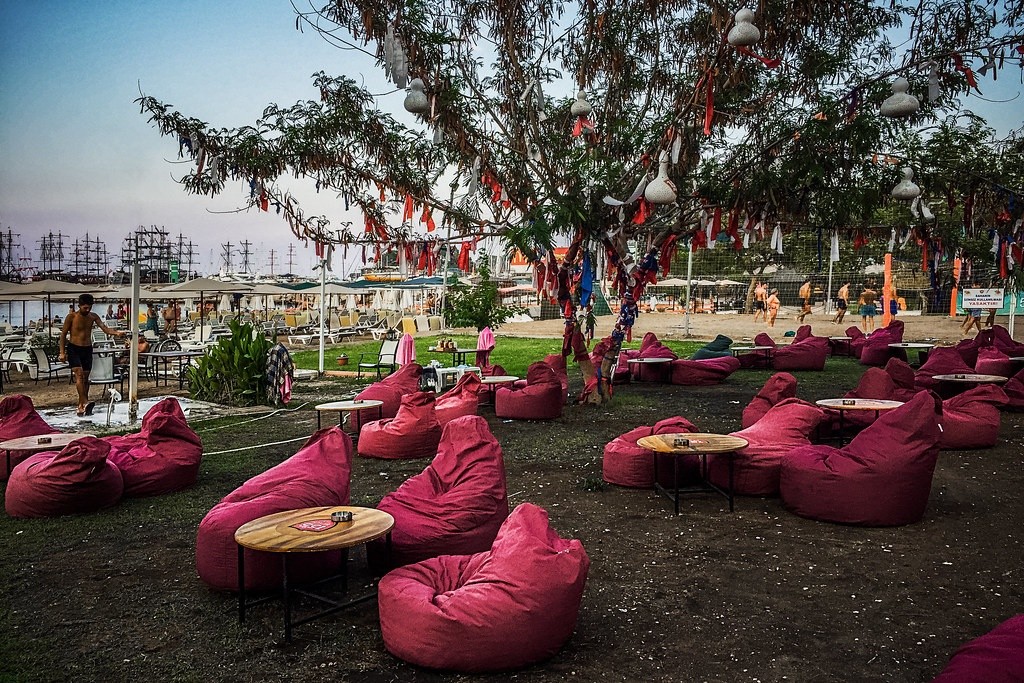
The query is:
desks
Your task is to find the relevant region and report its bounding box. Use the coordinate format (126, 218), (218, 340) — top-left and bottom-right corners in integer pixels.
(888, 343), (934, 366)
(234, 506), (395, 642)
(478, 376), (519, 413)
(436, 367), (482, 393)
(620, 348), (631, 355)
(731, 346), (773, 371)
(92, 348), (130, 366)
(829, 337), (852, 358)
(139, 351), (205, 390)
(637, 433), (749, 516)
(427, 349), (491, 383)
(627, 358), (673, 387)
(603, 320), (1024, 527)
(932, 374), (1008, 397)
(1009, 357), (1024, 361)
(816, 399), (905, 449)
(315, 400), (383, 441)
(0, 433), (97, 483)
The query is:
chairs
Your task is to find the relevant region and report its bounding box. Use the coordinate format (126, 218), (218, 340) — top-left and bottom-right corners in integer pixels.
(0, 308), (590, 671)
(931, 613), (1024, 683)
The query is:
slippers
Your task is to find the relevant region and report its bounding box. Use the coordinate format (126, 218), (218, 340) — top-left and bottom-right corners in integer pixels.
(85, 401), (95, 417)
(77, 411), (84, 416)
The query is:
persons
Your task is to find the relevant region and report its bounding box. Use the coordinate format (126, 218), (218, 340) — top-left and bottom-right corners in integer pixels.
(958, 281), (1000, 335)
(2, 293), (212, 417)
(677, 280), (907, 335)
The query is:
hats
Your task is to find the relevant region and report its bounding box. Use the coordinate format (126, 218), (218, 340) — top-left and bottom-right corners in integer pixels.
(771, 288), (776, 293)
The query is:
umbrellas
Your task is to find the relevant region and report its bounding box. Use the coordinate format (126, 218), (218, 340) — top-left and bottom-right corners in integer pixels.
(608, 279), (750, 314)
(0, 276), (536, 337)
